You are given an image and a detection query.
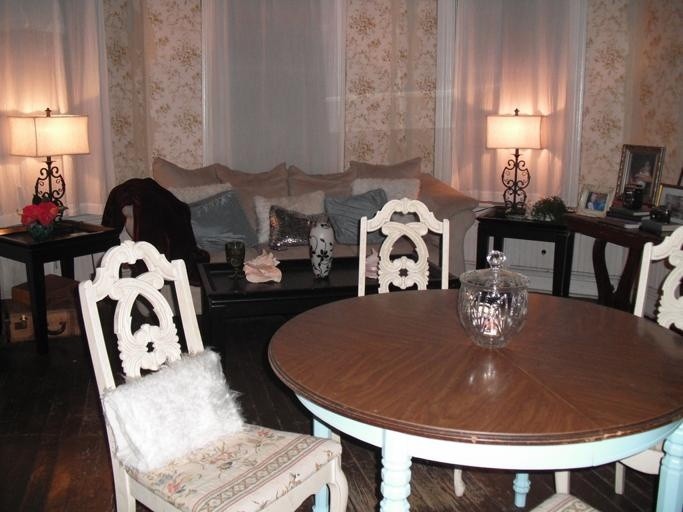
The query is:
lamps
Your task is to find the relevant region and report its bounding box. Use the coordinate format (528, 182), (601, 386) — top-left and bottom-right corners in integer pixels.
(486, 108), (542, 219)
(6, 108), (90, 241)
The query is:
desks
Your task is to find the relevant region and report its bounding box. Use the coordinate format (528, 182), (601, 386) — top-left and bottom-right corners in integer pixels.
(475, 205), (575, 297)
(0, 219), (120, 368)
(267, 289), (683, 512)
(561, 212), (683, 312)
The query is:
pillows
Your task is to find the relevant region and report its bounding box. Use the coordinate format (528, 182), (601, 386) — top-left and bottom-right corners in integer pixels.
(154, 157), (425, 252)
(100, 348), (245, 475)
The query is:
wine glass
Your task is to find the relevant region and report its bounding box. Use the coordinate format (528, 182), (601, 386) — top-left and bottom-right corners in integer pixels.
(223, 240), (248, 280)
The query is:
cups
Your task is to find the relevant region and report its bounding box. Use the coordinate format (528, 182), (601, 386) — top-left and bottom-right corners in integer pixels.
(458, 251), (530, 349)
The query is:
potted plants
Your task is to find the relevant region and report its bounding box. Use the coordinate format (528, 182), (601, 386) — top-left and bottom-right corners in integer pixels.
(531, 195), (569, 221)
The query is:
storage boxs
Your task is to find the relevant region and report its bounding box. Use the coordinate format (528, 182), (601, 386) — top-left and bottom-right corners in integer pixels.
(1, 274), (82, 343)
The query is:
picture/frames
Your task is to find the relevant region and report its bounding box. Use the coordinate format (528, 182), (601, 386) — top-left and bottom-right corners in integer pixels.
(575, 183), (616, 218)
(614, 144), (667, 210)
(654, 183), (683, 225)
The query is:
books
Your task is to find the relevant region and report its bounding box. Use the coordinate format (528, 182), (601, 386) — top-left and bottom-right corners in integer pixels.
(595, 206), (683, 238)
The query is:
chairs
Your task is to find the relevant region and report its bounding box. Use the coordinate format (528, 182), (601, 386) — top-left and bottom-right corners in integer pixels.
(359, 196), (466, 499)
(77, 240), (350, 512)
(529, 493), (599, 512)
(554, 225), (683, 493)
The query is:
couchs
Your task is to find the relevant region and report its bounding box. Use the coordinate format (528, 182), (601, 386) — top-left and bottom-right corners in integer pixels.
(116, 175), (478, 320)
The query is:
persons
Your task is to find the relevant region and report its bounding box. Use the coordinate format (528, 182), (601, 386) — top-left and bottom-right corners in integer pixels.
(586, 194), (596, 209)
